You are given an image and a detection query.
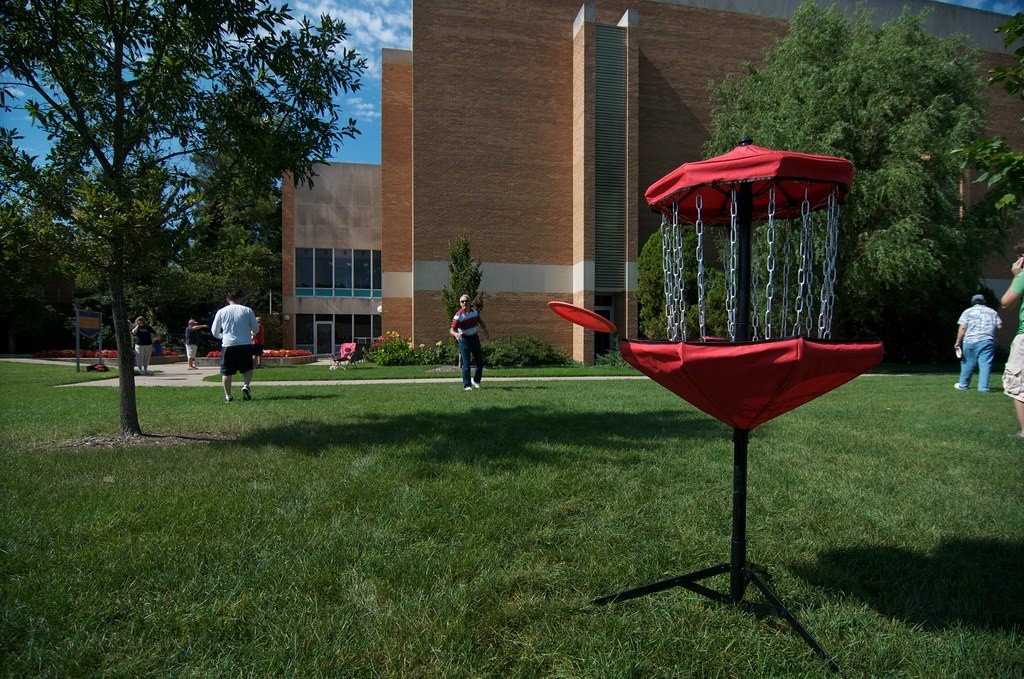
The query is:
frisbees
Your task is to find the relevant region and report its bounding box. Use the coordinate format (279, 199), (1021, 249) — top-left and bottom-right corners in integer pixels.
(956, 346), (963, 360)
(545, 300), (618, 333)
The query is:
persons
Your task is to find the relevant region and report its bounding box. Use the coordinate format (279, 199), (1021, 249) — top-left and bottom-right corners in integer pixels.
(952, 294), (1003, 392)
(211, 288), (259, 403)
(450, 295), (489, 391)
(1001, 240), (1024, 440)
(128, 316), (264, 374)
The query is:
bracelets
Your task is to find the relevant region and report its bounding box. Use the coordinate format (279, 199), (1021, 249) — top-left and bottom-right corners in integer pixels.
(484, 328), (487, 329)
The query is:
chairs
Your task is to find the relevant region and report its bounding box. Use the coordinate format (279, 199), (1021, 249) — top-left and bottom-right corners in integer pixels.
(327, 352), (359, 371)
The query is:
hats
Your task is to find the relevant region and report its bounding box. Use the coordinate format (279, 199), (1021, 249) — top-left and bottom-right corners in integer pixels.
(971, 294), (985, 302)
(189, 319), (198, 325)
(256, 317), (261, 322)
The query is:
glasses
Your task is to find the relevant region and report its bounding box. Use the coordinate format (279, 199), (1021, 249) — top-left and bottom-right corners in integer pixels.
(461, 300), (470, 303)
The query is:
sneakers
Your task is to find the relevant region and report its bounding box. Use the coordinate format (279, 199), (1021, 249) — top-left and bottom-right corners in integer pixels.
(241, 385), (251, 400)
(225, 395), (233, 403)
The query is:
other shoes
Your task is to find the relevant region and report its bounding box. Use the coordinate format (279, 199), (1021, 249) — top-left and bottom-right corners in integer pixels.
(1008, 430), (1024, 439)
(464, 387), (472, 390)
(256, 365), (263, 368)
(954, 383), (967, 390)
(471, 379), (480, 388)
(144, 371), (148, 375)
(139, 370), (143, 374)
(188, 367), (198, 370)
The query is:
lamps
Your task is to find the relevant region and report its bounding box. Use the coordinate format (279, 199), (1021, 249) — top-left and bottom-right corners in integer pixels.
(284, 314), (290, 321)
(377, 306), (382, 316)
